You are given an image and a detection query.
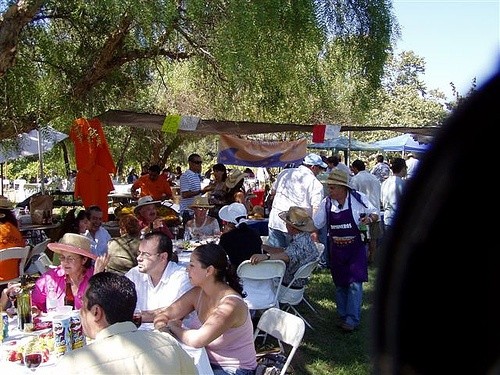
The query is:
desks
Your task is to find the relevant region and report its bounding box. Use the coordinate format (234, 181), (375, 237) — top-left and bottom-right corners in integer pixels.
(20, 223), (62, 231)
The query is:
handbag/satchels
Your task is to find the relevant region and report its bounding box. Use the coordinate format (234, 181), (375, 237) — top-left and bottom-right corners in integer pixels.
(29, 195), (53, 224)
(255, 353), (295, 375)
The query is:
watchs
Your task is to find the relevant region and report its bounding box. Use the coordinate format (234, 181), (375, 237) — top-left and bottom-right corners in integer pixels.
(266, 253), (271, 260)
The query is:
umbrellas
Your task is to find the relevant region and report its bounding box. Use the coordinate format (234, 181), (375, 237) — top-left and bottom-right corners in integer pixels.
(370, 133), (432, 159)
(0, 124), (69, 197)
(307, 135), (381, 166)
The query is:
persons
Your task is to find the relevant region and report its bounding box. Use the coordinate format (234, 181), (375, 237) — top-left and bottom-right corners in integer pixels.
(0, 70), (500, 375)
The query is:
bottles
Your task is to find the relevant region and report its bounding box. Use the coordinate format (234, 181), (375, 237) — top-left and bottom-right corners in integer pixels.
(221, 220), (229, 233)
(41, 211), (52, 225)
(24, 206), (29, 214)
(359, 213), (369, 243)
(16, 275), (33, 330)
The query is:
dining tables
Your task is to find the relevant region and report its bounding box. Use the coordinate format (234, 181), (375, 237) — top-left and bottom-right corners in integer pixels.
(172, 240), (227, 268)
(0, 314), (216, 375)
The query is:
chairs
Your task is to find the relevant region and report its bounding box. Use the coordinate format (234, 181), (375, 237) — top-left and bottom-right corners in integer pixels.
(24, 238), (51, 269)
(236, 235), (326, 375)
(0, 246), (30, 285)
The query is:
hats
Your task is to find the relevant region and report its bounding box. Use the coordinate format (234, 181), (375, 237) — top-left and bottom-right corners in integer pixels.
(320, 169), (352, 189)
(0, 197), (14, 209)
(47, 232), (96, 260)
(134, 196), (161, 214)
(187, 197), (215, 209)
(407, 153), (413, 157)
(302, 152), (328, 168)
(225, 169), (249, 189)
(219, 202), (248, 225)
(278, 206), (315, 232)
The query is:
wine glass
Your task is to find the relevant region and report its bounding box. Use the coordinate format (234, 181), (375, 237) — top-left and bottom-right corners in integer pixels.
(172, 227), (179, 242)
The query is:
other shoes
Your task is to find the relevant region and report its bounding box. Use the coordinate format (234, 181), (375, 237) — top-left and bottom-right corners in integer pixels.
(334, 318), (354, 330)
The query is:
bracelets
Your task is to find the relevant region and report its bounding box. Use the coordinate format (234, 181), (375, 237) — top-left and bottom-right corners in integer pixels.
(199, 190), (203, 195)
(368, 217), (373, 223)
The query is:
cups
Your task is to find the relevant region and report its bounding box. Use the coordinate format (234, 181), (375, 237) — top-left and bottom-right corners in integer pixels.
(248, 216), (254, 220)
(57, 305), (74, 316)
(132, 310), (142, 327)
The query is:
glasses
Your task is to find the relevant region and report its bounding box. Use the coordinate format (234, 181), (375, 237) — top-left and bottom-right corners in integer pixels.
(57, 255), (80, 262)
(192, 160), (202, 164)
(136, 251), (156, 257)
(196, 207), (208, 211)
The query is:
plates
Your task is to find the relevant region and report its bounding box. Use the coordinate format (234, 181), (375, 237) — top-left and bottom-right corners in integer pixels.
(246, 220), (256, 223)
(257, 218), (264, 220)
